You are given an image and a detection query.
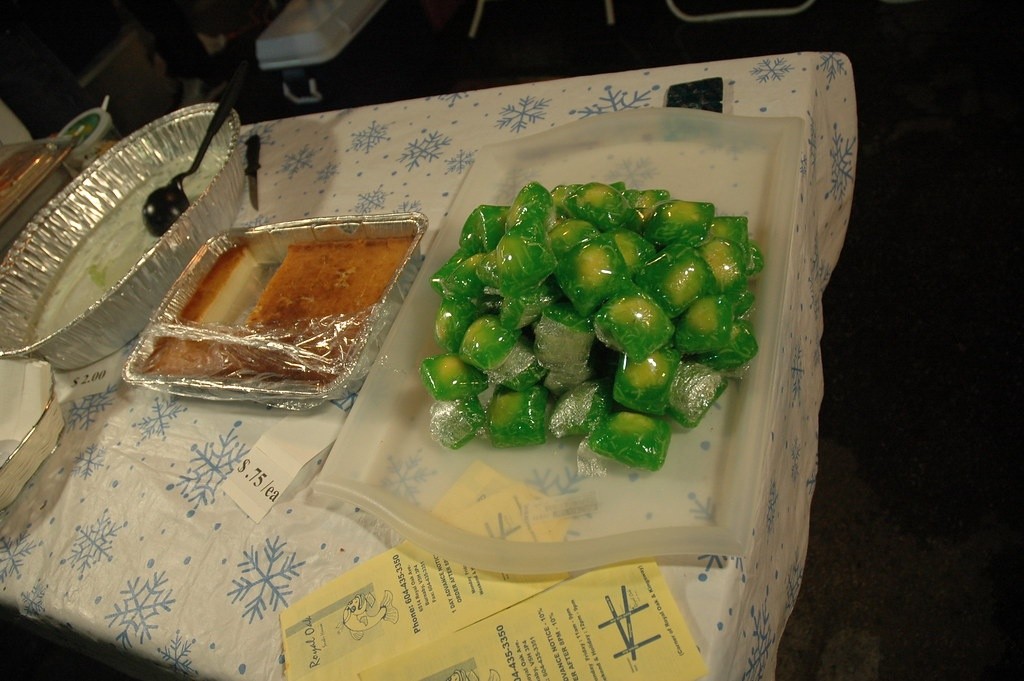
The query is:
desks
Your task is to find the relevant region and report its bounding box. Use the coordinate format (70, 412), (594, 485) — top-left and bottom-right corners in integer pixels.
(0, 50), (858, 681)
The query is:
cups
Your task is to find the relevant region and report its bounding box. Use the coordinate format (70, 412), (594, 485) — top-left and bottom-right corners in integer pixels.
(58, 108), (121, 174)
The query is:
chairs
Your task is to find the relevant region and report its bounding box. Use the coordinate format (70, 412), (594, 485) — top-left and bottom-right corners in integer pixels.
(663, 0), (817, 64)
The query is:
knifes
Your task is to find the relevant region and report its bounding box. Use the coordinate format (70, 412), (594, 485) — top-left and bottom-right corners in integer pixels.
(245, 135), (260, 211)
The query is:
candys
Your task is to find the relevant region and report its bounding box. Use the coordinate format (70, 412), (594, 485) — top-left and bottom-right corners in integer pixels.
(419, 180), (764, 465)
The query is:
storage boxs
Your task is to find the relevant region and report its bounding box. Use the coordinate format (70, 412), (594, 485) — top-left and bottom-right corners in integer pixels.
(246, 0), (434, 117)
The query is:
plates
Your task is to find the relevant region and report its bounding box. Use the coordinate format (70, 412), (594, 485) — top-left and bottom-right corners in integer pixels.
(314, 107), (802, 573)
